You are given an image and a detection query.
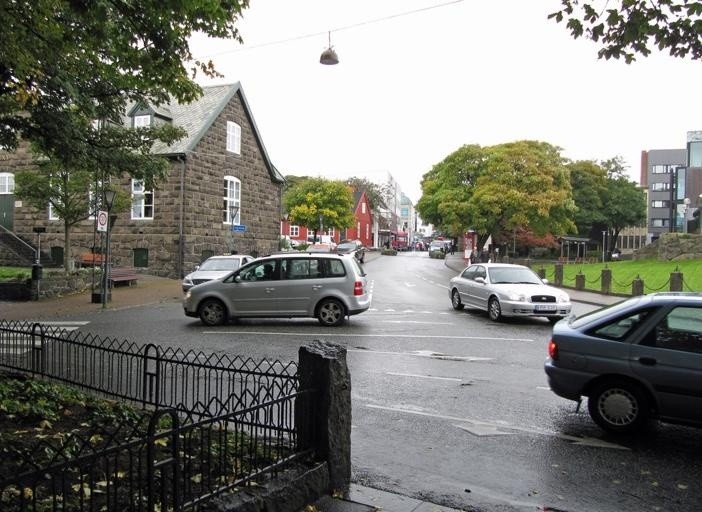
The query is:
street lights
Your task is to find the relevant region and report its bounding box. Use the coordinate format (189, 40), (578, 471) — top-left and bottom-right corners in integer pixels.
(227, 207), (240, 252)
(104, 175), (116, 310)
(683, 198), (690, 236)
(695, 193), (702, 208)
(601, 230), (607, 263)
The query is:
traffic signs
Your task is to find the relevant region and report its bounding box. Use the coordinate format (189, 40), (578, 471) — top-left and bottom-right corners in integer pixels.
(233, 224), (246, 231)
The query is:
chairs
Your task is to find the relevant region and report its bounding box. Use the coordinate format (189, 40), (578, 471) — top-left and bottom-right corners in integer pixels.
(631, 319), (656, 347)
(272, 265), (285, 279)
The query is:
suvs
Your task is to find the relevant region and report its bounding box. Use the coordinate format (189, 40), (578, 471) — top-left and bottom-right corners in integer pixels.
(183, 252), (371, 325)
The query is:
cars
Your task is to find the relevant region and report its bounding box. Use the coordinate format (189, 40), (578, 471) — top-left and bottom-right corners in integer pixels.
(428, 239), (454, 258)
(304, 241), (336, 254)
(448, 262), (573, 322)
(544, 290), (702, 436)
(332, 238), (365, 262)
(181, 253), (256, 296)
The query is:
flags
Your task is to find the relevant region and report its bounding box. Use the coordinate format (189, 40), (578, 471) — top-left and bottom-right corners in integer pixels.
(464, 232), (475, 259)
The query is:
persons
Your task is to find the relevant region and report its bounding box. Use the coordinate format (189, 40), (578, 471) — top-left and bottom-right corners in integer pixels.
(478, 246), (491, 263)
(470, 246), (479, 264)
(250, 264), (274, 282)
(450, 243), (455, 254)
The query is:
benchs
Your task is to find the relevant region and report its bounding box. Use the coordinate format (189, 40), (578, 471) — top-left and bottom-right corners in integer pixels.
(108, 268), (144, 289)
(75, 253), (112, 268)
